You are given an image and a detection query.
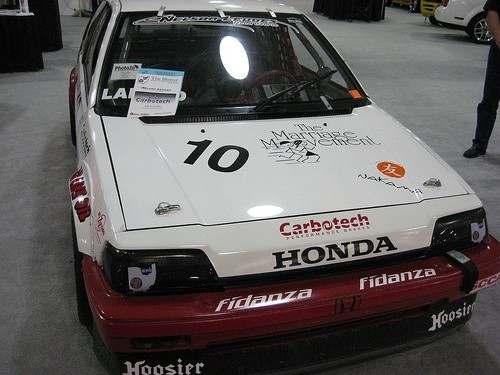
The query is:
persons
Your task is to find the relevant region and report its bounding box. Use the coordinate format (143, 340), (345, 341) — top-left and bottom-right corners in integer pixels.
(463, 1), (500, 158)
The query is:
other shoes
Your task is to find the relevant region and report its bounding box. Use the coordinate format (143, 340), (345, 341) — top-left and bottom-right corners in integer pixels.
(463, 139), (487, 158)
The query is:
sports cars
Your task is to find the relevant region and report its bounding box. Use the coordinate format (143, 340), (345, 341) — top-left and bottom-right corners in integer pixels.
(66, 0), (499, 375)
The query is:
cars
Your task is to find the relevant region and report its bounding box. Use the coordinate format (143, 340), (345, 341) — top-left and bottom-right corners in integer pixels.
(433, 0), (494, 45)
